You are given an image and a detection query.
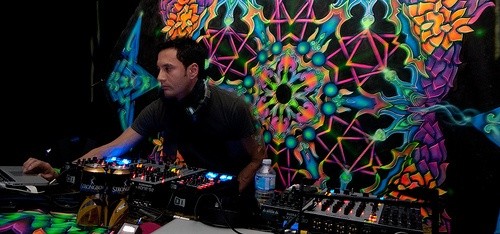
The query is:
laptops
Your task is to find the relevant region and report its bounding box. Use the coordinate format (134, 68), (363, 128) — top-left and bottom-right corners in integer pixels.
(0, 166), (49, 186)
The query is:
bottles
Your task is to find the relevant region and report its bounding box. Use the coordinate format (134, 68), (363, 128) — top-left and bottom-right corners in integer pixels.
(255, 159), (276, 206)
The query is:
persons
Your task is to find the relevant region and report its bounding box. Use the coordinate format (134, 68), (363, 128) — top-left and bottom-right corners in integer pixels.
(21, 38), (269, 196)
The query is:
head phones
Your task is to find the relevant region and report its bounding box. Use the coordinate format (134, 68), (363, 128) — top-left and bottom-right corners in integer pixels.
(185, 79), (211, 122)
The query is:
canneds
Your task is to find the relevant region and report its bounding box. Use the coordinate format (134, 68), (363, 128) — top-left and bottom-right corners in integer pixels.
(103, 166), (131, 227)
(76, 164), (107, 229)
